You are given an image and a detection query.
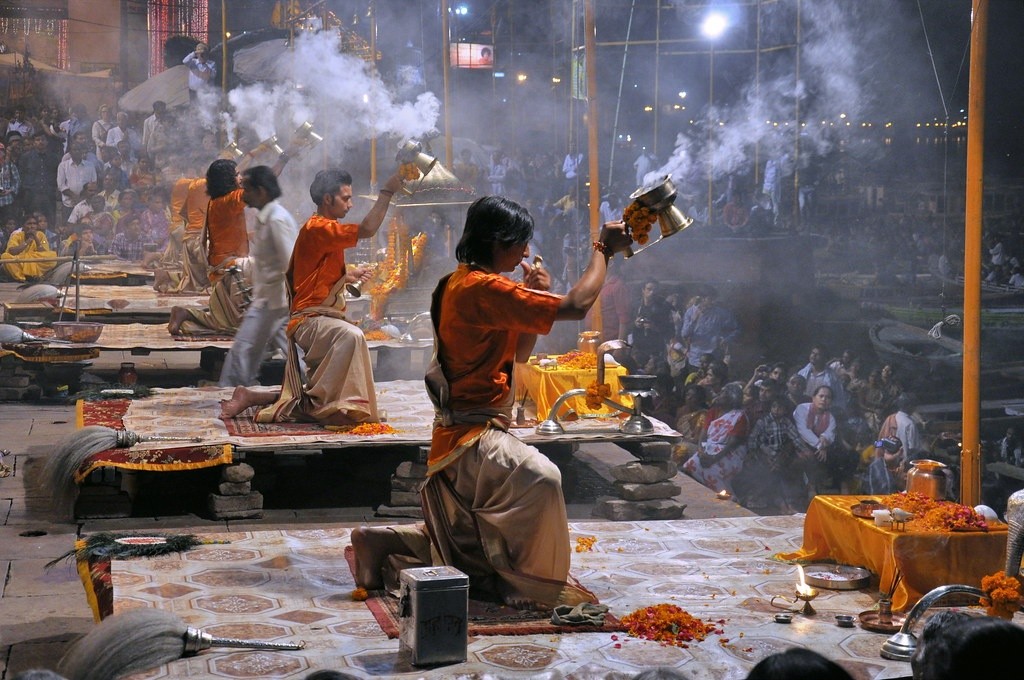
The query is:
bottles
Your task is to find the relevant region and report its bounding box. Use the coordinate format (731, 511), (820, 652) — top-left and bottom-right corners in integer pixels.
(906, 459), (949, 501)
(577, 331), (601, 354)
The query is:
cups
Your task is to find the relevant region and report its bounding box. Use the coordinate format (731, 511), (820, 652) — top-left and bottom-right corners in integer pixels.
(859, 500), (879, 514)
(516, 407), (524, 425)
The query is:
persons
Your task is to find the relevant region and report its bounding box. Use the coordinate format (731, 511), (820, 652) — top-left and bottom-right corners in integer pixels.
(748, 614), (1024, 680)
(349, 194), (636, 611)
(0, 44), (1024, 500)
(479, 47), (491, 64)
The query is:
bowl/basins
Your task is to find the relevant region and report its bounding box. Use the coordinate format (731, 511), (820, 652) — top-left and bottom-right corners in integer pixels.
(616, 375), (657, 391)
(774, 615), (792, 623)
(835, 615), (854, 627)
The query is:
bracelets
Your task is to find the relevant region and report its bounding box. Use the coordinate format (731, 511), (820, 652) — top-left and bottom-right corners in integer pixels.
(279, 152), (290, 162)
(37, 245), (42, 248)
(379, 189), (394, 196)
(24, 242), (28, 247)
(592, 240), (615, 260)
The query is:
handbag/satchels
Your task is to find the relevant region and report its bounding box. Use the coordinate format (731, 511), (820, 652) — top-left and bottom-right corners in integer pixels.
(671, 355), (688, 387)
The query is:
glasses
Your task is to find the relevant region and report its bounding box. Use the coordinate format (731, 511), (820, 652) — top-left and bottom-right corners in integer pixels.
(0, 148), (5, 151)
(882, 369), (892, 374)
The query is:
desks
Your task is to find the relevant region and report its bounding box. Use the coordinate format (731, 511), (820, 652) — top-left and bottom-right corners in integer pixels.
(506, 353), (629, 422)
(778, 496), (1015, 617)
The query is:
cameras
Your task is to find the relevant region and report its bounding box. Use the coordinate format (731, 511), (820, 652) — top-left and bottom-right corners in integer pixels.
(638, 317), (646, 324)
(201, 52), (209, 58)
(874, 437), (900, 452)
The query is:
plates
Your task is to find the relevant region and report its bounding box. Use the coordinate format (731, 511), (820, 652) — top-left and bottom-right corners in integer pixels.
(851, 504), (892, 519)
(858, 610), (907, 630)
